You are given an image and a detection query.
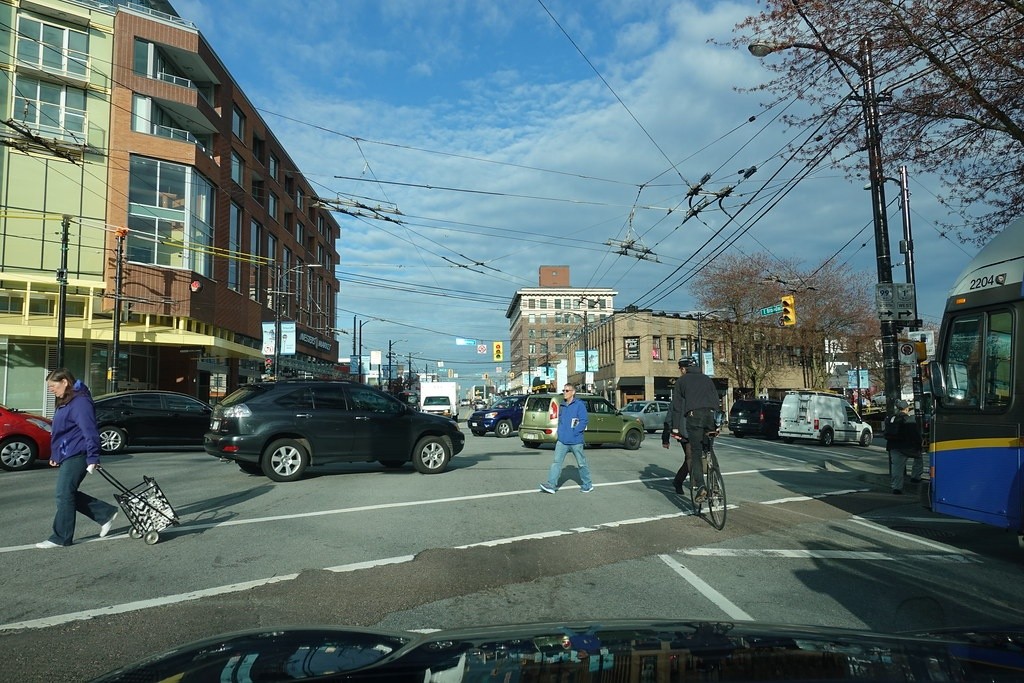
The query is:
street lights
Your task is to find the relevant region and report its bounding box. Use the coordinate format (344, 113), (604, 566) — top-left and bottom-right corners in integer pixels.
(275, 264), (322, 379)
(555, 311), (588, 395)
(863, 166), (924, 481)
(408, 352), (424, 390)
(529, 341), (549, 394)
(748, 38), (906, 474)
(359, 317), (385, 384)
(695, 309), (726, 374)
(388, 339), (408, 395)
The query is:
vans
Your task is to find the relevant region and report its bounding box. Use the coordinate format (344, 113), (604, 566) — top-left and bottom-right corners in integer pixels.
(777, 390), (873, 448)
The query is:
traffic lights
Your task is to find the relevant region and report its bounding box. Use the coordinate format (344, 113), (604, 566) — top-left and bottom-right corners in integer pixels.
(511, 373), (514, 379)
(781, 296), (796, 326)
(485, 375), (488, 380)
(494, 342), (503, 361)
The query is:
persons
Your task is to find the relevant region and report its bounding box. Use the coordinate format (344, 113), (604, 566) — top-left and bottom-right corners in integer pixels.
(569, 633), (603, 660)
(662, 389), (712, 495)
(36, 368), (119, 549)
(539, 383), (593, 493)
(677, 356), (719, 503)
(885, 400), (923, 493)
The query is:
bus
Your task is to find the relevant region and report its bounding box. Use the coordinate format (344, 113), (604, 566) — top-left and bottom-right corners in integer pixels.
(928, 217), (1024, 562)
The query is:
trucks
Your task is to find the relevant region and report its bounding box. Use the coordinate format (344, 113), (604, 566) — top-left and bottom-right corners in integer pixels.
(472, 384), (497, 408)
(419, 381), (460, 420)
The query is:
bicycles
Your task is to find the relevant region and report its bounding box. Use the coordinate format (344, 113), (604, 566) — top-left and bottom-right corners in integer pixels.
(678, 431), (726, 530)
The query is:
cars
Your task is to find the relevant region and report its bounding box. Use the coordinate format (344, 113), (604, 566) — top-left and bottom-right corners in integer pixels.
(0, 404), (52, 471)
(873, 391), (886, 406)
(461, 398), (470, 406)
(92, 391), (214, 456)
(476, 402), (487, 410)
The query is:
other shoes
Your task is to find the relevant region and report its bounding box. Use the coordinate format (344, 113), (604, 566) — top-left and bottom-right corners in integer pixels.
(712, 486), (721, 501)
(911, 477), (922, 482)
(672, 479), (684, 494)
(893, 488), (903, 495)
(695, 487), (708, 503)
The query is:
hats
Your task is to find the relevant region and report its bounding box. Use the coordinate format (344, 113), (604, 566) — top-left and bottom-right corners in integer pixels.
(896, 400), (911, 409)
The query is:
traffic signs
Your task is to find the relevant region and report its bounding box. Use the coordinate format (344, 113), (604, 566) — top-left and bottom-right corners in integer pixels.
(877, 283), (916, 321)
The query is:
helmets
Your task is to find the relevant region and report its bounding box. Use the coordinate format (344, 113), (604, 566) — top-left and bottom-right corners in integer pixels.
(678, 356), (696, 368)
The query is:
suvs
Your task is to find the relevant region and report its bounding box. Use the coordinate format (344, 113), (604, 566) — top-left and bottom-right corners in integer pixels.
(398, 392), (418, 411)
(202, 379), (464, 482)
(467, 392), (645, 450)
(728, 400), (782, 438)
(618, 401), (670, 434)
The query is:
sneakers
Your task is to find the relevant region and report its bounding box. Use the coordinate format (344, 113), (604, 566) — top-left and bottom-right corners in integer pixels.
(541, 484), (555, 493)
(36, 540), (63, 549)
(100, 512), (119, 536)
(580, 486), (594, 493)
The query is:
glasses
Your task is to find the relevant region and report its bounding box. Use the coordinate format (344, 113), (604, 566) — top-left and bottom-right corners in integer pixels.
(563, 390), (573, 393)
